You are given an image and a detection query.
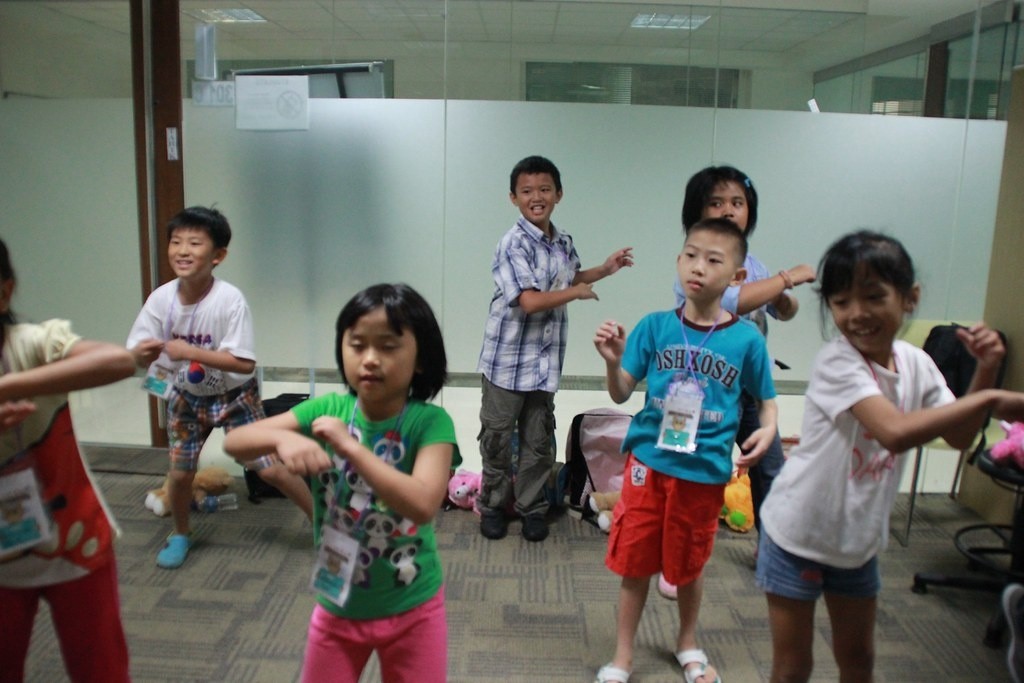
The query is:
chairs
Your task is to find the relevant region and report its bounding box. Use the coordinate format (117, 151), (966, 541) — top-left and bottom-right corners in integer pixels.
(890, 319), (1007, 546)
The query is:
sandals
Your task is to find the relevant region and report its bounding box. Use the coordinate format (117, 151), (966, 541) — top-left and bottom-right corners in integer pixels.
(156, 529), (195, 568)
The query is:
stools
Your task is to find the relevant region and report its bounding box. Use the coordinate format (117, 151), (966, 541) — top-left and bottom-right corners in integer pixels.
(911, 450), (1024, 650)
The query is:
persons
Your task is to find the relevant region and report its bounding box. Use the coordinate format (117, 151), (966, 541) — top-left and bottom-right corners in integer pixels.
(226, 284), (462, 683)
(657, 166), (817, 599)
(475, 156), (633, 543)
(758, 231), (1023, 683)
(0, 239), (137, 683)
(128, 207), (314, 569)
(595, 219), (778, 683)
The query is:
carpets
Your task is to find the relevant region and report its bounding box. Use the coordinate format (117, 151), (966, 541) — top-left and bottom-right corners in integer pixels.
(81, 444), (168, 476)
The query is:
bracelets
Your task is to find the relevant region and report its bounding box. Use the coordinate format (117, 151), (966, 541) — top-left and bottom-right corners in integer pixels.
(779, 270), (793, 289)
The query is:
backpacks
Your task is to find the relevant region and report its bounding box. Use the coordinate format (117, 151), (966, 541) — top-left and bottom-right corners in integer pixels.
(566, 407), (636, 509)
(244, 393), (311, 500)
(922, 322), (1008, 432)
(546, 461), (567, 523)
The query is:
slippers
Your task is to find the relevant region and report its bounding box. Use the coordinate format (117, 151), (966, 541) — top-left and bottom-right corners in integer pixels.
(673, 643), (721, 683)
(594, 661), (634, 683)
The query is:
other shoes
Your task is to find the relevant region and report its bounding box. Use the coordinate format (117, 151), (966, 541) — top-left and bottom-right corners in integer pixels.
(1001, 584), (1024, 683)
(658, 571), (678, 599)
(750, 545), (758, 566)
(480, 514), (509, 540)
(521, 513), (549, 542)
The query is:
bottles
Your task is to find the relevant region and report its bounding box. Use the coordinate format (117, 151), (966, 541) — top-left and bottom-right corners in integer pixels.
(191, 493), (239, 513)
